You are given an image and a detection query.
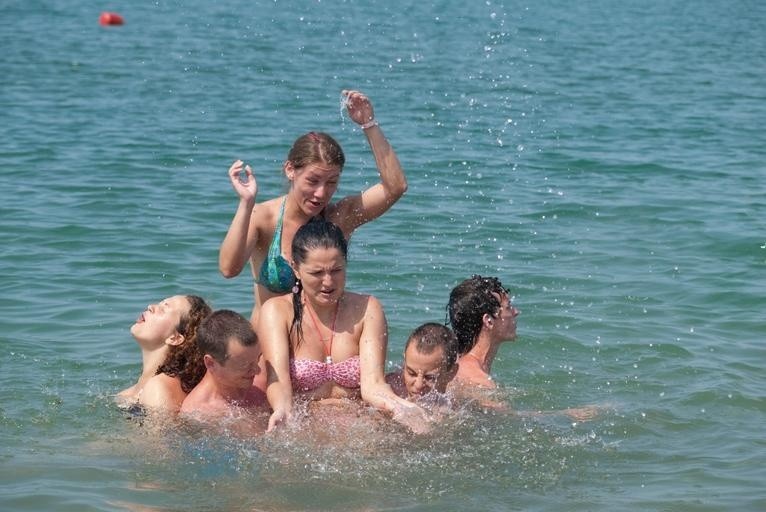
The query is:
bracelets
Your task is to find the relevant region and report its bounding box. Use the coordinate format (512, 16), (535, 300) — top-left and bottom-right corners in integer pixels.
(360, 120), (376, 129)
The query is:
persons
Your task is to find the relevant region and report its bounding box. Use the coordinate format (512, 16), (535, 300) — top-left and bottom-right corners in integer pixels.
(260, 214), (434, 438)
(218, 89), (410, 335)
(445, 273), (599, 420)
(385, 322), (460, 409)
(177, 309), (272, 439)
(113, 294), (212, 420)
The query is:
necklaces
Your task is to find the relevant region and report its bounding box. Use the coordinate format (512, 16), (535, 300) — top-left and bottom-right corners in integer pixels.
(303, 298), (340, 365)
